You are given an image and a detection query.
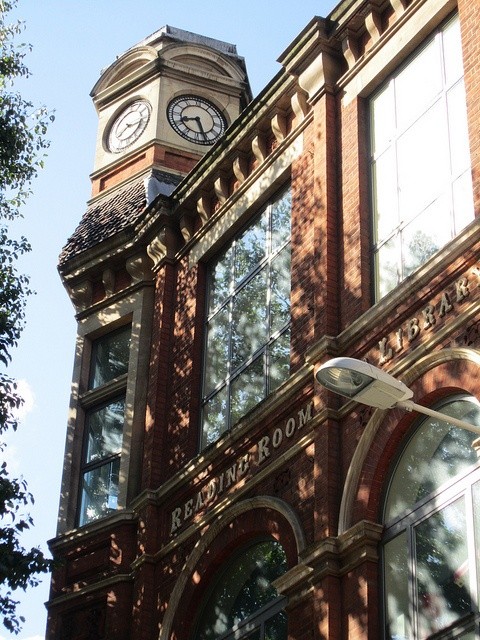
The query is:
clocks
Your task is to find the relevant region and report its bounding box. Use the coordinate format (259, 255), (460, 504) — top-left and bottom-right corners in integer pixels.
(106, 97), (152, 154)
(166, 94), (228, 145)
